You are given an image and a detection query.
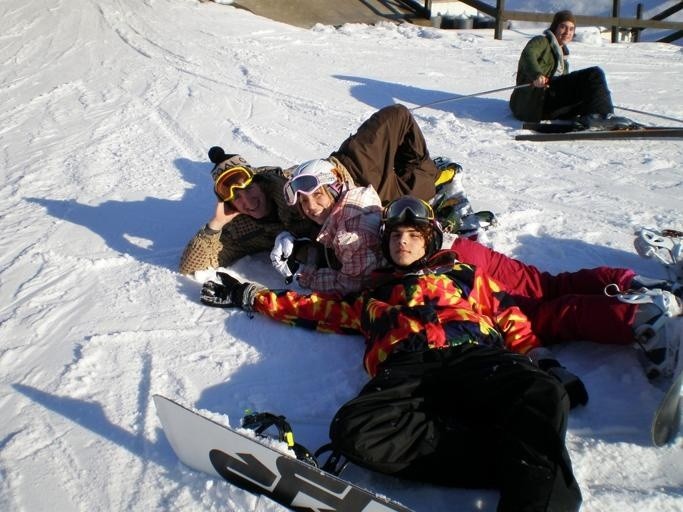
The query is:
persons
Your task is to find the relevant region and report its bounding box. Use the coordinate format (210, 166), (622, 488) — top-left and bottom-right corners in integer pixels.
(270, 159), (682, 387)
(198, 196), (589, 511)
(508, 11), (637, 127)
(181, 103), (462, 278)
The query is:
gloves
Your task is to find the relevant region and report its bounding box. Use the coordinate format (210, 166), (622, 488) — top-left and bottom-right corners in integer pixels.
(270, 232), (301, 283)
(200, 270), (257, 313)
(525, 347), (589, 411)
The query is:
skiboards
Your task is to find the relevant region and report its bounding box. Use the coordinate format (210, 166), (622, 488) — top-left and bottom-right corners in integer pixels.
(516, 123), (683, 140)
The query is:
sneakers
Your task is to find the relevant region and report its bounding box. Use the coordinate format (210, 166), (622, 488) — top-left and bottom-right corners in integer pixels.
(617, 274), (683, 384)
(435, 163), (462, 186)
(586, 115), (635, 129)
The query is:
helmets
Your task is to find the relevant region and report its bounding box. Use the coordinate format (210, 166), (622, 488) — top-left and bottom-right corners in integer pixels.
(291, 160), (342, 198)
(378, 197), (443, 261)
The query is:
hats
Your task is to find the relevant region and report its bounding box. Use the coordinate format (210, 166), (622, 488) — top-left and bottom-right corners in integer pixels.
(208, 146), (253, 202)
(550, 11), (577, 38)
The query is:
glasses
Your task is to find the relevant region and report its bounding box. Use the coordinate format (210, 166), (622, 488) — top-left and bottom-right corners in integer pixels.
(214, 167), (254, 201)
(283, 174), (320, 207)
(381, 195), (434, 224)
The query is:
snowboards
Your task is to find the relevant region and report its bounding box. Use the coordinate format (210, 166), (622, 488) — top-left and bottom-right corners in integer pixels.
(153, 394), (417, 512)
(433, 155), (479, 241)
(652, 376), (682, 448)
